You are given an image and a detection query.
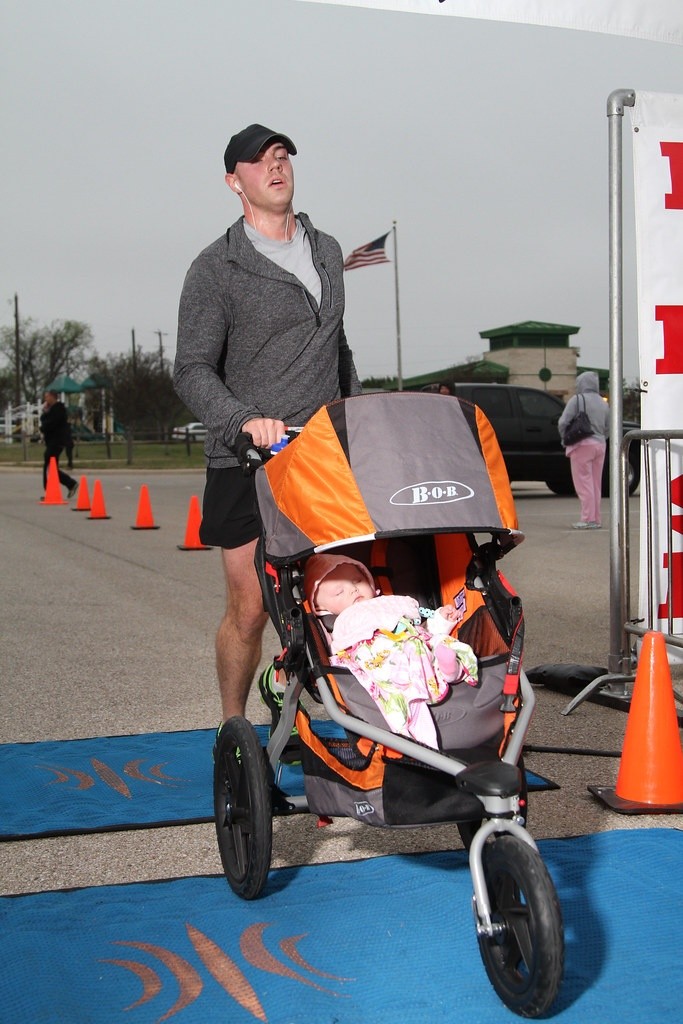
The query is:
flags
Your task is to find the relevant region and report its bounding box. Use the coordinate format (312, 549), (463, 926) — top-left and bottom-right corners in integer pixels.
(344, 232), (391, 272)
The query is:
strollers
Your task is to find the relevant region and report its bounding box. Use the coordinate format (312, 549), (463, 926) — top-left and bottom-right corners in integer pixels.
(213, 389), (568, 1021)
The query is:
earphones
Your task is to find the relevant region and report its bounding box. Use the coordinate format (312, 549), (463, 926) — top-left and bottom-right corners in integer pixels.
(234, 181), (243, 193)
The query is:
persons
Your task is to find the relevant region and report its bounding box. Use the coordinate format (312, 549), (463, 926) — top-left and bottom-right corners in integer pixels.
(305, 554), (478, 750)
(557, 372), (610, 530)
(38, 389), (79, 501)
(438, 379), (456, 396)
(173, 124), (362, 765)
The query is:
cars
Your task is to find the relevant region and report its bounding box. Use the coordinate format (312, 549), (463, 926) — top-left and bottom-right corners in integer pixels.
(171, 423), (207, 443)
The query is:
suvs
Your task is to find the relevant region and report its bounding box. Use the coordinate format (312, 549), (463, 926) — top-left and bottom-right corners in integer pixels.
(425, 383), (641, 497)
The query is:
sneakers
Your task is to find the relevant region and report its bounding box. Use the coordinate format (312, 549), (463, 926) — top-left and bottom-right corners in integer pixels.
(573, 522), (601, 528)
(257, 663), (311, 765)
(212, 721), (295, 811)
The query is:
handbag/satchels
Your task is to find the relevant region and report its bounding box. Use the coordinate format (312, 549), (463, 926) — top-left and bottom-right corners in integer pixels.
(564, 393), (594, 446)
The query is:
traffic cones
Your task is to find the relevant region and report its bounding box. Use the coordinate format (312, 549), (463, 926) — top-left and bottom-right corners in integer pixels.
(71, 476), (91, 511)
(176, 495), (213, 550)
(86, 480), (111, 519)
(587, 629), (683, 816)
(37, 457), (69, 505)
(130, 485), (161, 530)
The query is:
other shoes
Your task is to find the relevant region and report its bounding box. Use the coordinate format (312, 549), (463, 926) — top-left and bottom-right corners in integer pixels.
(40, 496), (44, 500)
(66, 482), (78, 498)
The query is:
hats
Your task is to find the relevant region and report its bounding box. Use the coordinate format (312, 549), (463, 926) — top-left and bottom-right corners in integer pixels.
(224, 124), (297, 173)
(304, 555), (375, 615)
